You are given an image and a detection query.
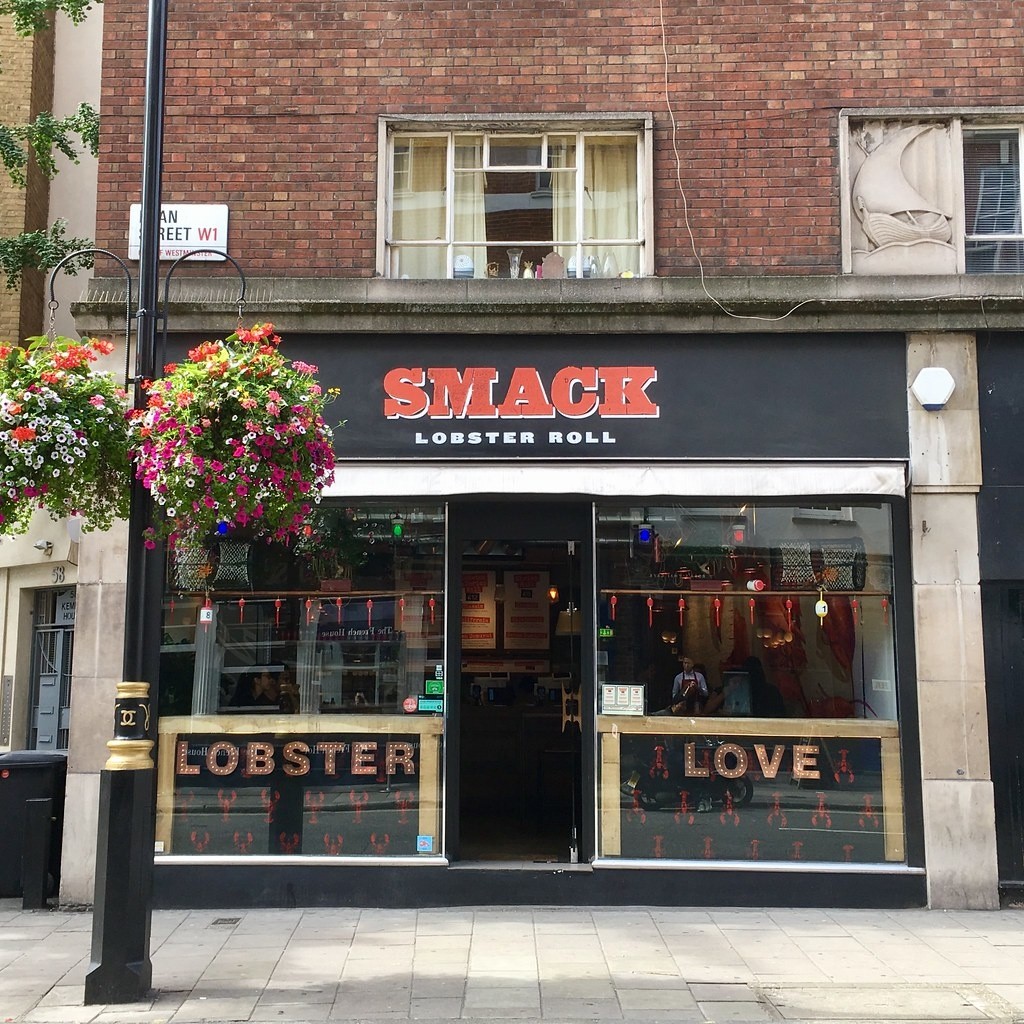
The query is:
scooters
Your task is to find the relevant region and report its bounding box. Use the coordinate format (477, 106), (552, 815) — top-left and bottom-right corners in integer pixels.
(618, 723), (754, 812)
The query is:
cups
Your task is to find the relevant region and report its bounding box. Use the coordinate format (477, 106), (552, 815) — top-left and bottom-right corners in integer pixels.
(602, 251), (619, 278)
(588, 255), (602, 278)
(506, 249), (523, 279)
(746, 580), (767, 592)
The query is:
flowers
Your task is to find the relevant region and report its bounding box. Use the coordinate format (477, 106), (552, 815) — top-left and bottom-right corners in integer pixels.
(0, 322), (336, 550)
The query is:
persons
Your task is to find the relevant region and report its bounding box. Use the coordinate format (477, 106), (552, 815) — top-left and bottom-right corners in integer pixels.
(651, 653), (794, 719)
(255, 660), (292, 712)
(225, 663), (276, 714)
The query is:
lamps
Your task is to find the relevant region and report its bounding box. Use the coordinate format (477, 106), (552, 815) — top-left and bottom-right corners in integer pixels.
(909, 338), (956, 410)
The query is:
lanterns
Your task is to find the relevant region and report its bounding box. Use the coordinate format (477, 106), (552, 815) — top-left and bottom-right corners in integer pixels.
(166, 597), (439, 625)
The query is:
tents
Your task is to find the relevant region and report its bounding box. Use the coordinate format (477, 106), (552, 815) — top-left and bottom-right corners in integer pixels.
(608, 593), (889, 620)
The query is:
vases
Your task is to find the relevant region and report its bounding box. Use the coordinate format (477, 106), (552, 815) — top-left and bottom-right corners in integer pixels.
(174, 547), (209, 592)
(211, 538), (251, 590)
(507, 248), (523, 278)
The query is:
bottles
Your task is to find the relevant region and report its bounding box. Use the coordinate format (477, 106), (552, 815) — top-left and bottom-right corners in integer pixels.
(279, 684), (301, 714)
